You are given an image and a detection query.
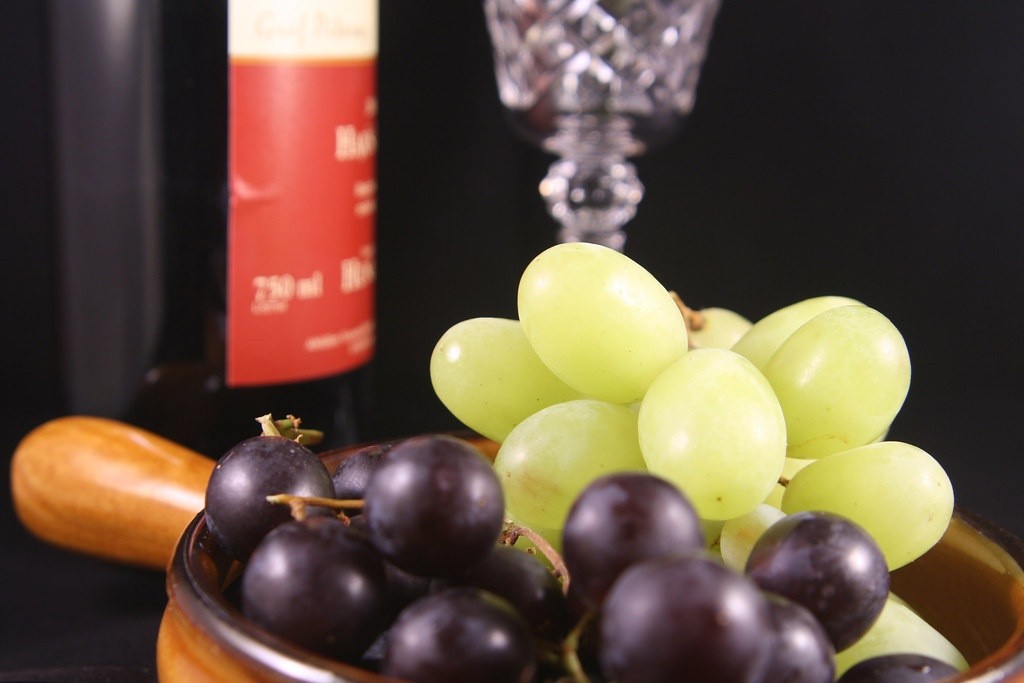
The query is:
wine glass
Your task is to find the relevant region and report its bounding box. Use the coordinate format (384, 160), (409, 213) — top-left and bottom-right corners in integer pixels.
(486, 1), (718, 262)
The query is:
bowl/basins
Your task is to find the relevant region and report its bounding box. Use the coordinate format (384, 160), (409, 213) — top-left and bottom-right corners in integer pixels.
(6, 406), (1024, 681)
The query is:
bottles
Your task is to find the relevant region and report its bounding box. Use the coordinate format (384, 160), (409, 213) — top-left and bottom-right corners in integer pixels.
(0, 0), (377, 481)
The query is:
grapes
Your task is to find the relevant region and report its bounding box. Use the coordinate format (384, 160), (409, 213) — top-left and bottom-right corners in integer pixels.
(204, 240), (973, 682)
